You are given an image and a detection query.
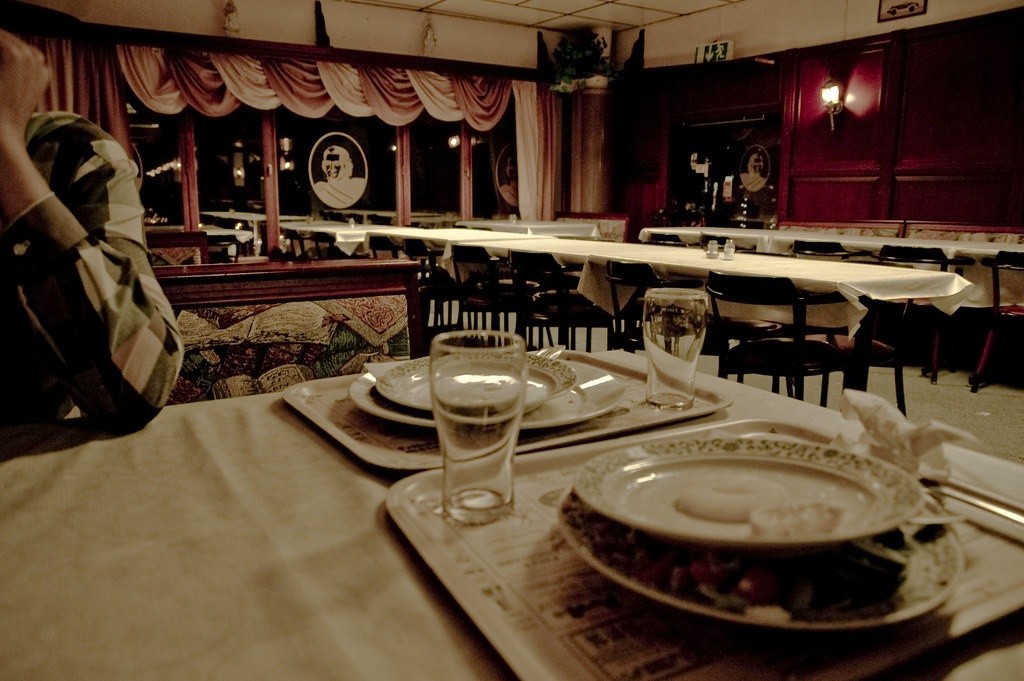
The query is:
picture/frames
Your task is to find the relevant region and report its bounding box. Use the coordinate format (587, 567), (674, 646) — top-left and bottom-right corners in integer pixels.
(876, 0), (928, 23)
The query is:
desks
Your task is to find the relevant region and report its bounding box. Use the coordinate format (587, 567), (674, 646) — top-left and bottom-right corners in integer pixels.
(456, 238), (731, 354)
(198, 210), (269, 255)
(638, 226), (778, 254)
(952, 243), (1023, 371)
(592, 246), (975, 402)
(765, 226), (958, 371)
(366, 225), (558, 284)
(274, 220), (373, 258)
(455, 219), (601, 242)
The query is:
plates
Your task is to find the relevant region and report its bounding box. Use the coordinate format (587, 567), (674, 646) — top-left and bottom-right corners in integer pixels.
(572, 428), (925, 552)
(375, 351), (578, 420)
(349, 357), (624, 430)
(553, 494), (971, 633)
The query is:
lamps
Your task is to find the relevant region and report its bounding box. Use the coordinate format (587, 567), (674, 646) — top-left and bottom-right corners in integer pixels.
(821, 73), (845, 132)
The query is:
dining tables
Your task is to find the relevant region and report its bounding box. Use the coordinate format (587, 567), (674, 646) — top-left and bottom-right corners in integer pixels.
(0, 352), (1023, 681)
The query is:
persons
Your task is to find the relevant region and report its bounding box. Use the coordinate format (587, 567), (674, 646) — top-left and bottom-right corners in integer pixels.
(0, 29), (185, 436)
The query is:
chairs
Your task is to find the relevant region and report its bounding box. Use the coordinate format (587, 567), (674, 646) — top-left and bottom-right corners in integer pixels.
(198, 214), (1024, 420)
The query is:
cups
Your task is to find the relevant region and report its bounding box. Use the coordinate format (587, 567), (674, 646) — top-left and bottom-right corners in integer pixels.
(431, 330), (527, 526)
(642, 287), (710, 411)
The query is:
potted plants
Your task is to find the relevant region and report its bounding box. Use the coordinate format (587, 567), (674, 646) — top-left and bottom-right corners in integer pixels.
(552, 32), (612, 90)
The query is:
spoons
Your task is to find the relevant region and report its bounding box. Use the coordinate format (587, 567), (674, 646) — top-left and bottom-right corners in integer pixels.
(538, 346), (567, 361)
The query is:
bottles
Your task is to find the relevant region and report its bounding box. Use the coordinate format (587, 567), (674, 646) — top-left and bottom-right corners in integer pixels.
(509, 213), (518, 224)
(705, 239), (719, 259)
(723, 239), (735, 260)
(348, 217), (355, 229)
